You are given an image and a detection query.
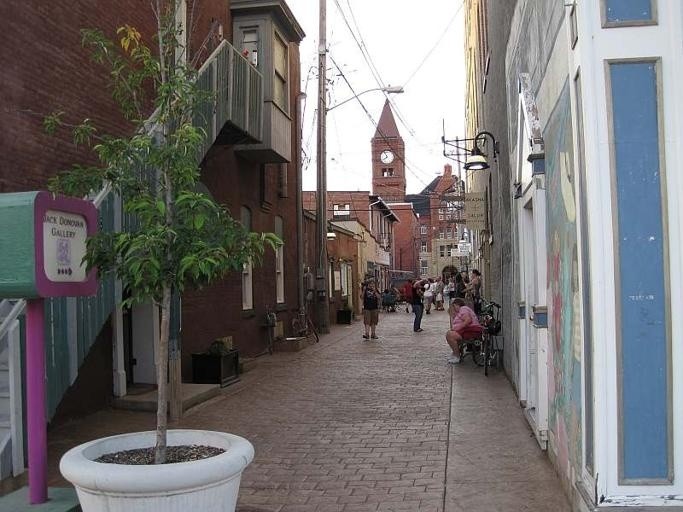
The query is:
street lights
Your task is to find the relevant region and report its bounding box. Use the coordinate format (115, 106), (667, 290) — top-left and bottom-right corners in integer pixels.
(326, 86), (404, 110)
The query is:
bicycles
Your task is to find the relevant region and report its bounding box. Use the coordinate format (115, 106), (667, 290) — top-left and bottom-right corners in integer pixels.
(289, 303), (320, 345)
(476, 297), (502, 377)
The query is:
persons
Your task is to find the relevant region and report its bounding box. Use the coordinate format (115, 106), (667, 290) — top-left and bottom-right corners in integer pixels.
(446, 298), (481, 363)
(381, 268), (481, 332)
(359, 278), (380, 339)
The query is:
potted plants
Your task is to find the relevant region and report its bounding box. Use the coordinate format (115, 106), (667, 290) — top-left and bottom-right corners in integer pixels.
(41, 1), (286, 512)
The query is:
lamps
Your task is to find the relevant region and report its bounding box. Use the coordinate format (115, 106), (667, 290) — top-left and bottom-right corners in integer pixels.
(463, 131), (499, 171)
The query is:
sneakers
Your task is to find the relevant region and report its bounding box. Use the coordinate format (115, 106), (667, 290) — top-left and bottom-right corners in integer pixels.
(363, 334), (378, 340)
(414, 328), (423, 332)
(447, 355), (461, 364)
